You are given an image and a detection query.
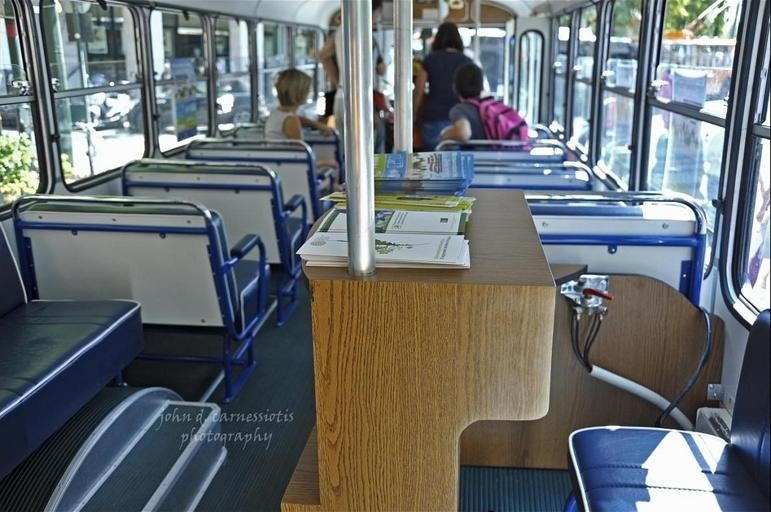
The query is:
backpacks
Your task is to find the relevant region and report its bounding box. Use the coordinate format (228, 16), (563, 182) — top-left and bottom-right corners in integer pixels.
(466, 96), (528, 150)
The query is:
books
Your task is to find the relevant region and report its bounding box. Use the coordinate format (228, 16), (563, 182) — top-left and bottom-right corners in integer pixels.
(293, 151), (477, 271)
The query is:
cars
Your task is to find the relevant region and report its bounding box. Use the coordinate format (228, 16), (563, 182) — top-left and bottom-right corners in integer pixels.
(92, 76), (267, 132)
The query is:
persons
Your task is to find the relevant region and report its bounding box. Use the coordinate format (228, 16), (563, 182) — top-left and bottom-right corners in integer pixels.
(438, 62), (491, 148)
(319, 0), (428, 183)
(264, 68), (344, 193)
(414, 21), (472, 151)
